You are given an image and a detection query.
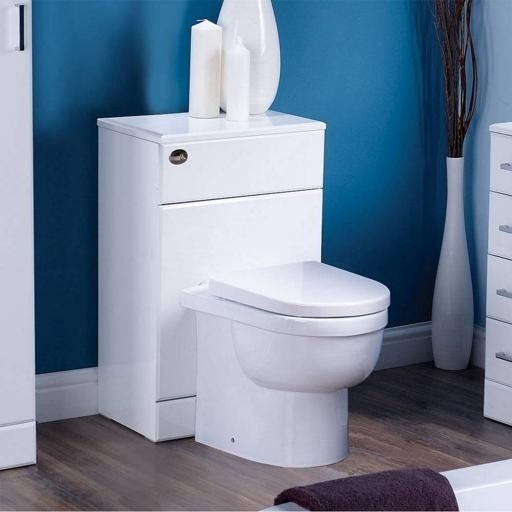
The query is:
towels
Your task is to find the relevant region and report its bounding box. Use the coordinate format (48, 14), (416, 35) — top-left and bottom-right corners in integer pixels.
(273, 467), (460, 511)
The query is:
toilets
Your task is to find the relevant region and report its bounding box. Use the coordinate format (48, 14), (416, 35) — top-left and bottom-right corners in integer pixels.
(96, 109), (391, 473)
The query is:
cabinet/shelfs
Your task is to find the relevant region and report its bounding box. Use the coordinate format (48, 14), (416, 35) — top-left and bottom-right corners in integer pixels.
(483, 122), (512, 427)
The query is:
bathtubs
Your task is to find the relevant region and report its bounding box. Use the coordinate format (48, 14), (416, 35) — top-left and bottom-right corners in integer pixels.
(258, 456), (511, 512)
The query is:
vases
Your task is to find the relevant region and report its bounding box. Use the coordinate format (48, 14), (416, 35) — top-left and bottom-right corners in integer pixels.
(216, 0), (281, 116)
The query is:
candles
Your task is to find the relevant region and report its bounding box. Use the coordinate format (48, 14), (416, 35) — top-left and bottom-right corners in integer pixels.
(188, 18), (222, 119)
(225, 41), (249, 121)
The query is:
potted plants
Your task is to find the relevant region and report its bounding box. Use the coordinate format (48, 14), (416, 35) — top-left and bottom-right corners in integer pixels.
(428, 0), (478, 371)
